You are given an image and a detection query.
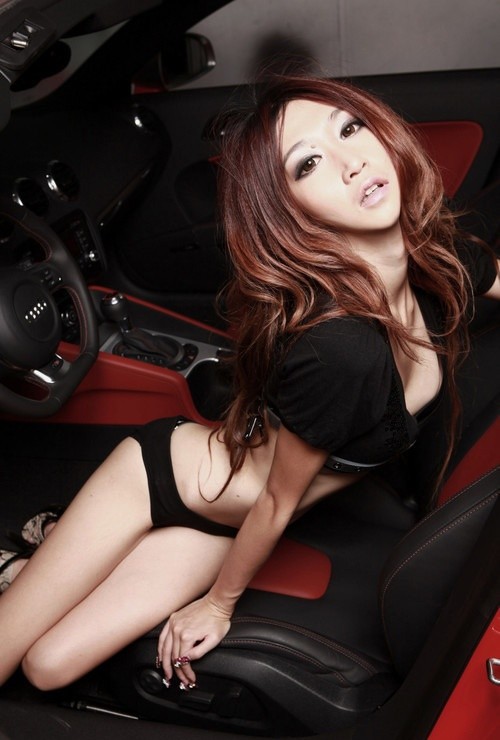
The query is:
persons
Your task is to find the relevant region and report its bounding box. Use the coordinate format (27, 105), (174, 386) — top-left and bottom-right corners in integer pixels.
(1, 71), (499, 691)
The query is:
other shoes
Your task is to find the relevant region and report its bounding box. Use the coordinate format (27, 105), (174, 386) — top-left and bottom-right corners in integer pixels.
(0, 550), (30, 593)
(16, 511), (59, 546)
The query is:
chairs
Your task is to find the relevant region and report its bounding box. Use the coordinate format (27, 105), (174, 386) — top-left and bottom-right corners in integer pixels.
(78, 392), (500, 740)
